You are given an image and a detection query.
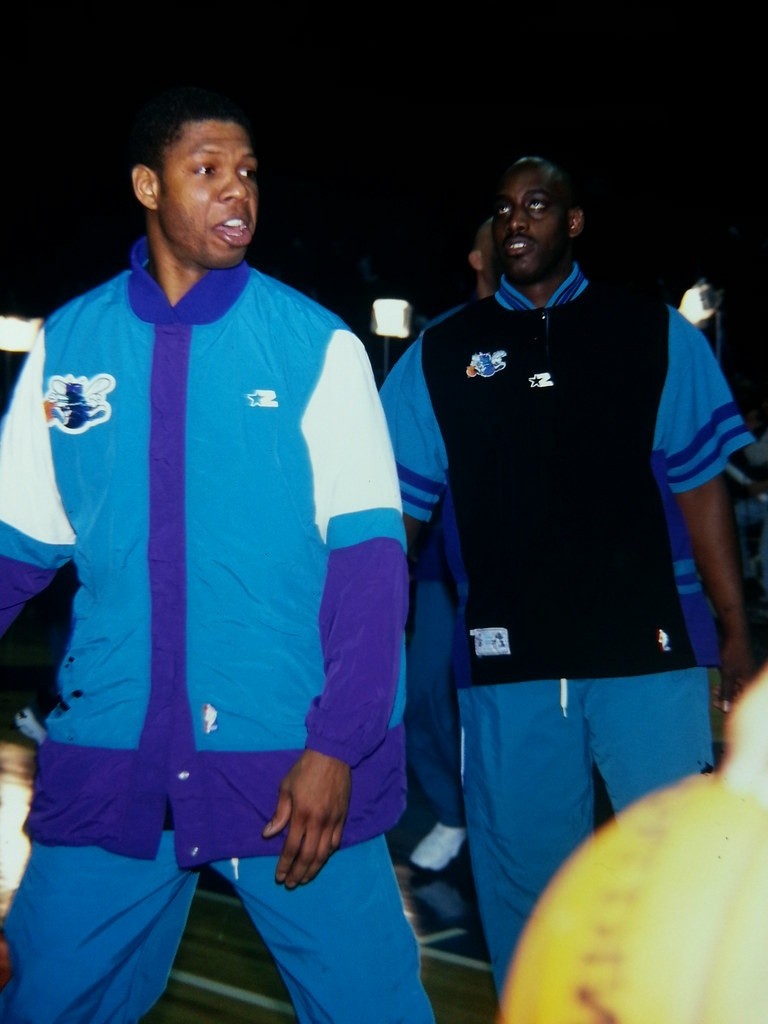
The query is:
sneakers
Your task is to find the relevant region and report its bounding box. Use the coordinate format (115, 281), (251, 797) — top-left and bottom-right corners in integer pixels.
(411, 822), (466, 871)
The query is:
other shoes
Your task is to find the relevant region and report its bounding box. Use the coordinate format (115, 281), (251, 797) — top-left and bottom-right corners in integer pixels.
(15, 702), (49, 748)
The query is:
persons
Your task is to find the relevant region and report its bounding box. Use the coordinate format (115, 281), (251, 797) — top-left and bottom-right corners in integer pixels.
(375, 160), (768, 1024)
(0, 86), (436, 1024)
